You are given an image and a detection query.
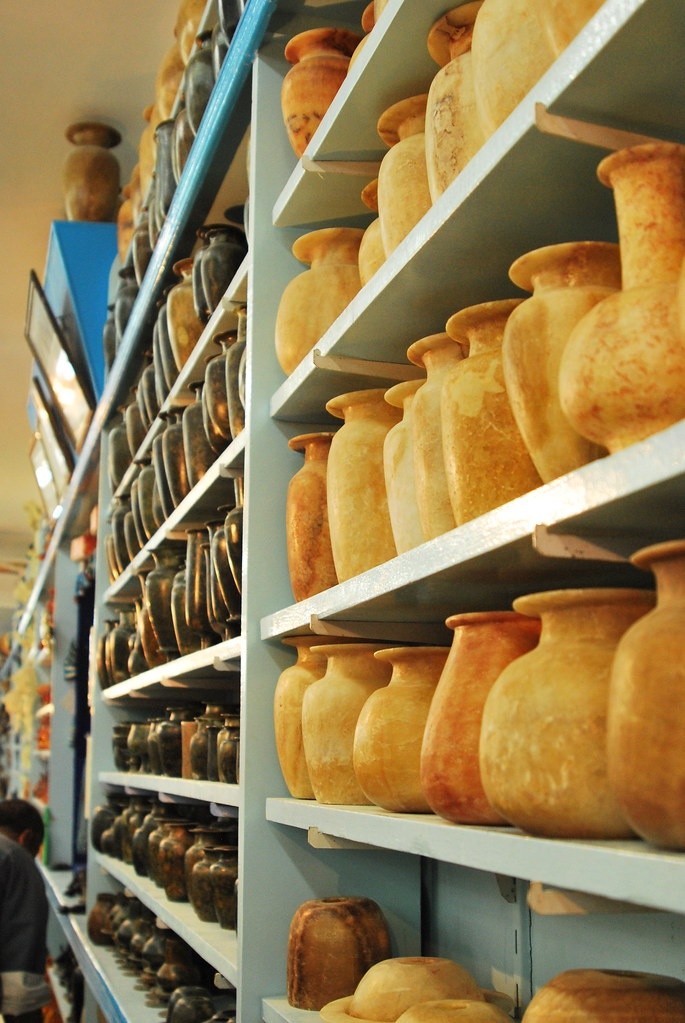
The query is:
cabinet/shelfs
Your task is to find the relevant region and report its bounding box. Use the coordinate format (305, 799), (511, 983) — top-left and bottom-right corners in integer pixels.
(1, 1), (684, 1023)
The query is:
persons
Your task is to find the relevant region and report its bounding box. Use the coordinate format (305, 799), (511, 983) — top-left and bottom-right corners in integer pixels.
(0, 799), (49, 1023)
